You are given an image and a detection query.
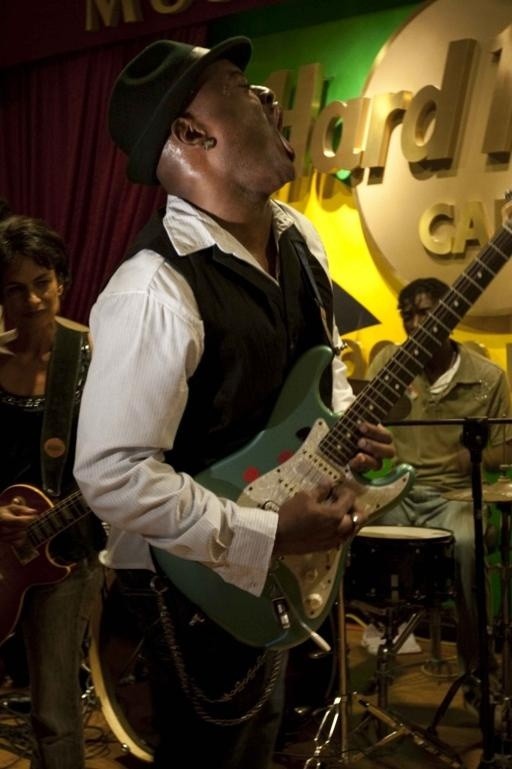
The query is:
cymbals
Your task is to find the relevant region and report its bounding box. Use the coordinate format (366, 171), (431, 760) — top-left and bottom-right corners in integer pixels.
(442, 476), (512, 503)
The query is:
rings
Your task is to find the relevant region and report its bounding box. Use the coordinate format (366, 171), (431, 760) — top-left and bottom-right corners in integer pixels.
(351, 512), (360, 531)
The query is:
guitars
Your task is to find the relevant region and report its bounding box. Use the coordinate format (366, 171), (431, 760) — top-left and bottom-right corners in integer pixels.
(1, 485), (92, 639)
(147, 203), (512, 648)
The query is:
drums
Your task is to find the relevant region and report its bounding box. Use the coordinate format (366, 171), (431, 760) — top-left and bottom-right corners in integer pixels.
(349, 524), (454, 622)
(91, 570), (347, 769)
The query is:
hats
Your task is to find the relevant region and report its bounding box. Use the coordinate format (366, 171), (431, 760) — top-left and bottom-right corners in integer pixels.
(107, 33), (253, 184)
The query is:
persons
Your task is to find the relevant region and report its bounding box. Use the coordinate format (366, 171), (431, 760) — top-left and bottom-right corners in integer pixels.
(0, 213), (107, 768)
(364, 276), (510, 723)
(72, 36), (399, 766)
